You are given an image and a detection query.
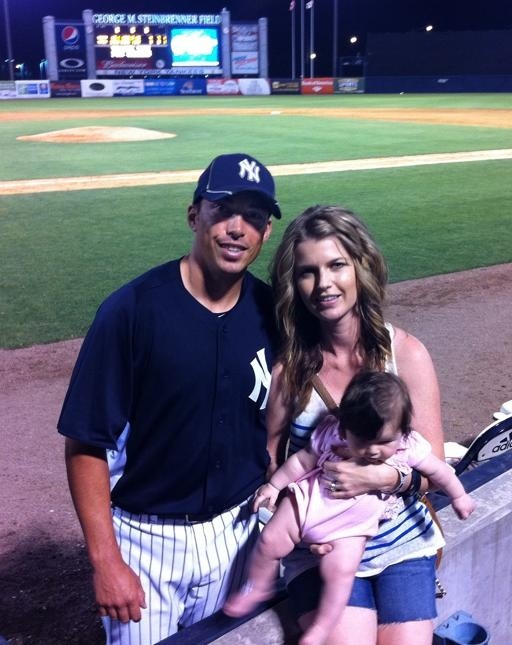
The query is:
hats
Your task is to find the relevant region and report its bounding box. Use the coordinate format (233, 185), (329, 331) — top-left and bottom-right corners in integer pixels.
(193, 153), (282, 220)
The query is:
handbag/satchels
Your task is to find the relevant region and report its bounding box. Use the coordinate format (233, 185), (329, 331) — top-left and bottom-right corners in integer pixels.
(419, 494), (444, 570)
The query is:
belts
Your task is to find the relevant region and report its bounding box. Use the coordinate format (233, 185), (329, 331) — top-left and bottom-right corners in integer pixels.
(157, 506), (226, 522)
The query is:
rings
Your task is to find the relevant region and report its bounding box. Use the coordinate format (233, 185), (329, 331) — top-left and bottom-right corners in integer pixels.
(329, 480), (337, 491)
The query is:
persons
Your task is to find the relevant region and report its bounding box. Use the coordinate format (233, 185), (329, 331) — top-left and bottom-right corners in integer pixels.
(221, 371), (474, 645)
(57, 154), (285, 645)
(264, 203), (446, 645)
